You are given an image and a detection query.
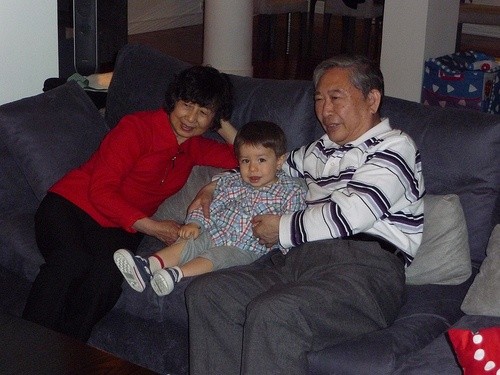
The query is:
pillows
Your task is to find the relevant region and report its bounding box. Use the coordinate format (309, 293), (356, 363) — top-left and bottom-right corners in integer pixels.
(404, 193), (472, 285)
(0, 79), (111, 202)
(448, 327), (500, 375)
(460, 222), (500, 318)
(151, 165), (227, 226)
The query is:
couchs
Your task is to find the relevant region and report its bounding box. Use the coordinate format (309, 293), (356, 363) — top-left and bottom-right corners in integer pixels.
(0, 44), (500, 375)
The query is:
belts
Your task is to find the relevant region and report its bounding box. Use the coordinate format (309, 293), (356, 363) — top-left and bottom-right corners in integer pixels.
(347, 233), (406, 265)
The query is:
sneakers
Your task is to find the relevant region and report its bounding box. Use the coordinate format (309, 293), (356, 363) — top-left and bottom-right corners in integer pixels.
(151, 268), (179, 297)
(113, 248), (149, 292)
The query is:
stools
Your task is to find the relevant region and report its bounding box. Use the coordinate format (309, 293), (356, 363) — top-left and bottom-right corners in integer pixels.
(420, 48), (500, 114)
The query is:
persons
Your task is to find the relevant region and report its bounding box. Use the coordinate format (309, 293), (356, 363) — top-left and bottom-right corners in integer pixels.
(113, 120), (308, 297)
(22, 66), (238, 345)
(185, 57), (427, 375)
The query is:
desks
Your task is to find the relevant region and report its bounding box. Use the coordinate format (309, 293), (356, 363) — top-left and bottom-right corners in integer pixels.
(43, 78), (107, 109)
(0, 315), (97, 375)
